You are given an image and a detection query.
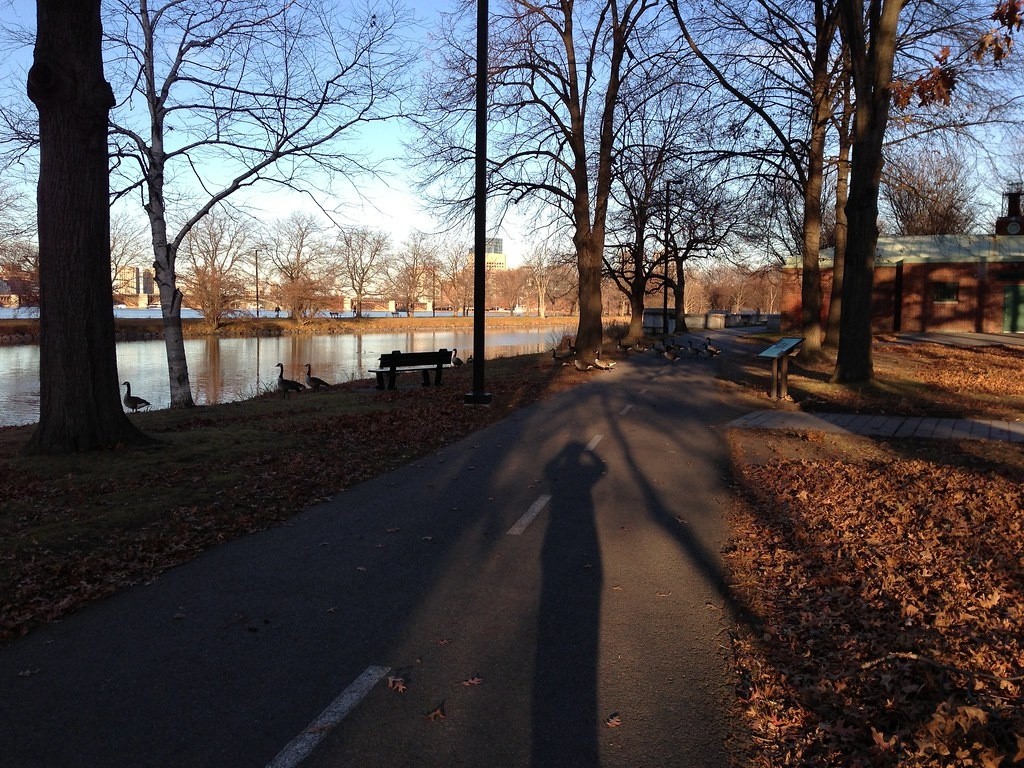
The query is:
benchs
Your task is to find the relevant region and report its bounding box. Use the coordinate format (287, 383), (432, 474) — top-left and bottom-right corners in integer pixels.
(368, 348), (453, 388)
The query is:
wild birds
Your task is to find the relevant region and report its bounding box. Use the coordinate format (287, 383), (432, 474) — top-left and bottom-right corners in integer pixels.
(635, 338), (686, 365)
(275, 363), (306, 399)
(702, 343), (721, 357)
(566, 338), (575, 351)
(452, 348), (463, 367)
(593, 350), (618, 372)
(562, 351), (577, 367)
(705, 337), (722, 353)
(122, 381), (152, 412)
(687, 341), (705, 360)
(616, 337), (632, 356)
(303, 364), (333, 393)
(549, 349), (565, 367)
(574, 359), (598, 372)
